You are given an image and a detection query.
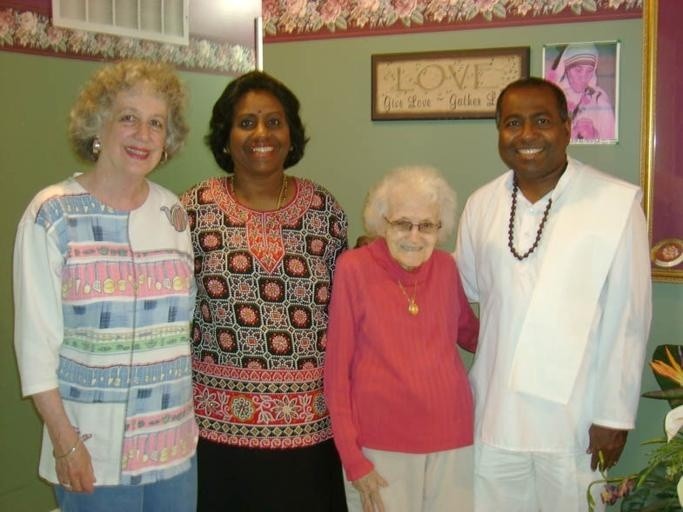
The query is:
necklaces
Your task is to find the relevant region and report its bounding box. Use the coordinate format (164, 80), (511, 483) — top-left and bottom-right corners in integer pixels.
(508, 161), (571, 260)
(396, 280), (421, 315)
(228, 172), (289, 211)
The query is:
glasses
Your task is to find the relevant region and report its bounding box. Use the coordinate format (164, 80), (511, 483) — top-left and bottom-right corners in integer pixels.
(385, 216), (441, 234)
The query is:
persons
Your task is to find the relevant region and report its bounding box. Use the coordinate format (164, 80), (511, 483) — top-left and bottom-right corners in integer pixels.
(450, 76), (656, 512)
(546, 45), (614, 139)
(12, 57), (202, 510)
(321, 163), (477, 512)
(181, 67), (347, 510)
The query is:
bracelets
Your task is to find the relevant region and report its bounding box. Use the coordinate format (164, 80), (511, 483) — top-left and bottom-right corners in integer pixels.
(52, 432), (82, 462)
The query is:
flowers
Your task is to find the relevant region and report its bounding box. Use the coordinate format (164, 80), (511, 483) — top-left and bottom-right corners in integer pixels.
(586, 346), (682, 511)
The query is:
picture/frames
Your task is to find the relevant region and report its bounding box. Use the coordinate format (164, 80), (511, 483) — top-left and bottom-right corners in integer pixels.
(369, 44), (531, 121)
(639, 0), (682, 284)
